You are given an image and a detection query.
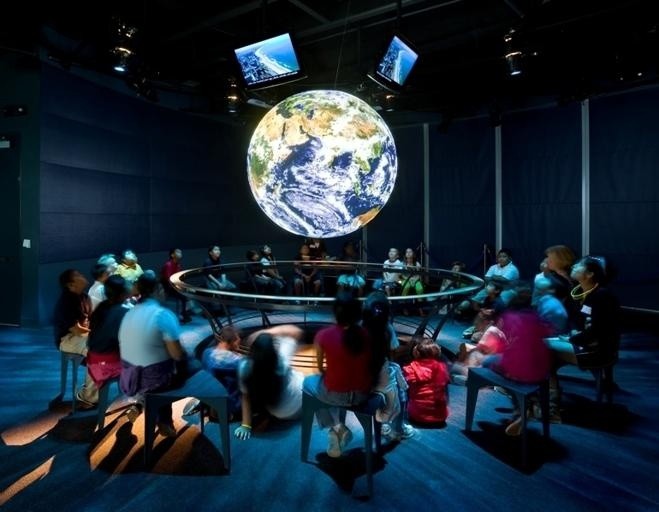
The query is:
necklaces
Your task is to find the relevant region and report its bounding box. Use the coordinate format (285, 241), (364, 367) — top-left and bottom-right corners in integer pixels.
(571, 283), (600, 300)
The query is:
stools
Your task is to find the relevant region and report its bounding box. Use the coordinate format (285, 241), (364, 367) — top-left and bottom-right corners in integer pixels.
(465, 367), (552, 460)
(98, 371), (121, 431)
(61, 352), (85, 396)
(144, 368), (231, 470)
(555, 359), (614, 406)
(301, 391), (405, 498)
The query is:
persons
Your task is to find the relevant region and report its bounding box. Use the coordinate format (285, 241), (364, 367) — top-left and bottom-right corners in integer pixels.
(431, 265), (470, 316)
(369, 291), (413, 439)
(478, 291), (557, 437)
(543, 243), (575, 299)
(53, 269), (100, 407)
(294, 244), (322, 305)
(99, 254), (120, 276)
(405, 338), (450, 428)
(471, 248), (519, 310)
(535, 256), (624, 426)
(88, 275), (130, 386)
(378, 247), (404, 312)
(261, 242), (287, 294)
(337, 243), (365, 298)
(88, 260), (113, 308)
(203, 327), (248, 426)
(201, 245), (235, 314)
(400, 247), (423, 316)
(120, 272), (205, 439)
(114, 250), (144, 282)
(532, 260), (550, 303)
(162, 245), (202, 323)
(246, 251), (273, 289)
(304, 292), (379, 457)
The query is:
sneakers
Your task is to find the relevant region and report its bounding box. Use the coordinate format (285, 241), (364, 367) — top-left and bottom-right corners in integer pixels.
(326, 428), (340, 458)
(127, 403), (144, 423)
(502, 406), (531, 438)
(391, 425), (418, 441)
(336, 426), (353, 447)
(379, 425), (388, 437)
(159, 419), (177, 439)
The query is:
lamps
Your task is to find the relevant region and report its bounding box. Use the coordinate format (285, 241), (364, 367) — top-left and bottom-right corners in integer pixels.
(503, 29), (537, 76)
(112, 21), (138, 73)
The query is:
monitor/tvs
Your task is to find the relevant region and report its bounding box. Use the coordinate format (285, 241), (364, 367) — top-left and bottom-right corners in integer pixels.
(367, 35), (419, 95)
(233, 32), (307, 92)
